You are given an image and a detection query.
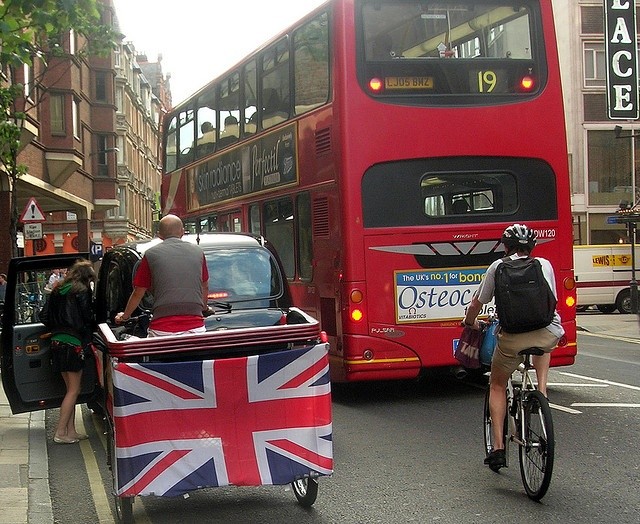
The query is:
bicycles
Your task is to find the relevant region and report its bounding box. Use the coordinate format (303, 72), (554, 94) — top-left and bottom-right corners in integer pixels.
(460, 315), (554, 500)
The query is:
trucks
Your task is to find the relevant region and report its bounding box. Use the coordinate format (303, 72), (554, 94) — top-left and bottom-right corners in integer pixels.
(573, 245), (640, 314)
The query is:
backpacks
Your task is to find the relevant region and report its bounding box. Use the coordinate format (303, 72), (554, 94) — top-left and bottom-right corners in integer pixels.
(495, 257), (557, 334)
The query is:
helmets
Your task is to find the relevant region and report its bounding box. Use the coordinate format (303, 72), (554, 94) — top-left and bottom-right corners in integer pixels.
(501, 223), (540, 247)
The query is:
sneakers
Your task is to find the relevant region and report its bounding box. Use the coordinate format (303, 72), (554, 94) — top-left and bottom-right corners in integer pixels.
(484, 447), (508, 466)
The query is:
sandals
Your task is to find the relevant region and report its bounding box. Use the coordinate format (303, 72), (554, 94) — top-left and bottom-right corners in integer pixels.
(54, 435), (89, 443)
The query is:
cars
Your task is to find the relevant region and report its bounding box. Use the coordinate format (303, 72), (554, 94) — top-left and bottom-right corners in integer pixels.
(0, 231), (294, 414)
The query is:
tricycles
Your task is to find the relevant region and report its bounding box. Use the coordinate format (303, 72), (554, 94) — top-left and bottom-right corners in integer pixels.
(91, 307), (334, 524)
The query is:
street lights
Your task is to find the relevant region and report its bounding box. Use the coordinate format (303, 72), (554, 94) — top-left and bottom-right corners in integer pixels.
(614, 125), (640, 138)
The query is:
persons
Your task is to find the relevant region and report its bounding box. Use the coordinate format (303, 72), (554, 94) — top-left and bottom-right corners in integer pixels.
(0, 274), (8, 304)
(114, 214), (215, 338)
(50, 259), (99, 445)
(45, 270), (60, 293)
(197, 121), (215, 139)
(58, 267), (67, 279)
(246, 89), (288, 124)
(224, 116), (237, 127)
(462, 223), (566, 465)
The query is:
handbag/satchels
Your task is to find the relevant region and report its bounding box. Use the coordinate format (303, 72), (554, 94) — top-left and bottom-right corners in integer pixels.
(455, 320), (485, 371)
(481, 322), (499, 366)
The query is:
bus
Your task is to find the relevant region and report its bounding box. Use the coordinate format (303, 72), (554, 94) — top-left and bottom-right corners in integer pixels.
(162, 0), (576, 382)
